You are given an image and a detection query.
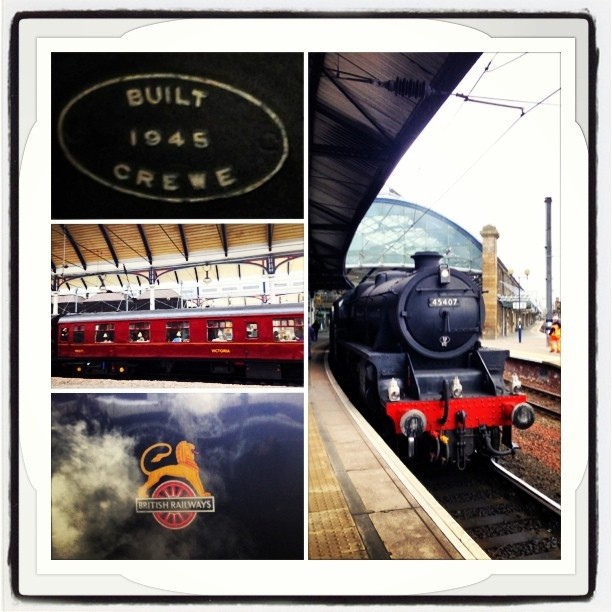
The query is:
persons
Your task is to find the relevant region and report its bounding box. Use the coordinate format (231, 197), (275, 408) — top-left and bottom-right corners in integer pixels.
(312, 320), (320, 341)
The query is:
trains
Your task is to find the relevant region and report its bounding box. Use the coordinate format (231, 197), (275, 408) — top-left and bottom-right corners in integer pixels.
(52, 302), (303, 385)
(328, 250), (538, 466)
